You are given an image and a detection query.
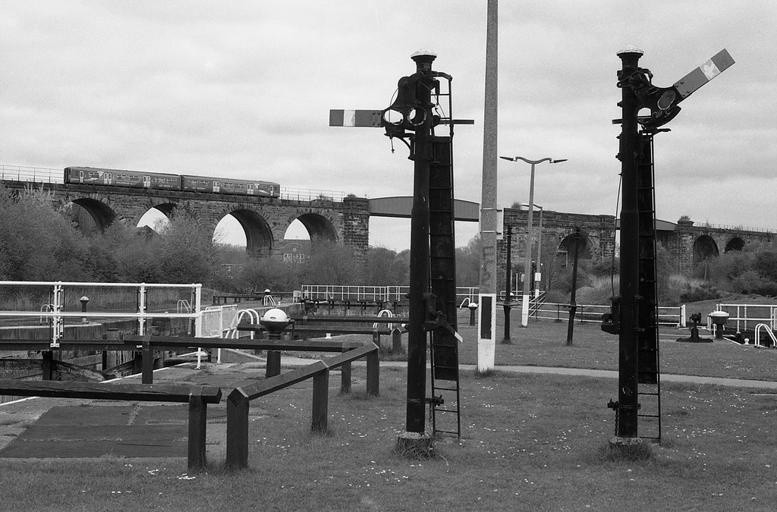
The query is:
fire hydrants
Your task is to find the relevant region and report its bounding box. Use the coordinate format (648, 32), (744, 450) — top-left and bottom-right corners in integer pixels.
(689, 312), (701, 341)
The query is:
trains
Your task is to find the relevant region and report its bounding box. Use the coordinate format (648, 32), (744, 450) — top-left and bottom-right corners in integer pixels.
(64, 166), (280, 199)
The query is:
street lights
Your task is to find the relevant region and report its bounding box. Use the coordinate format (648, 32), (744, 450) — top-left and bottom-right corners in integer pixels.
(500, 156), (568, 327)
